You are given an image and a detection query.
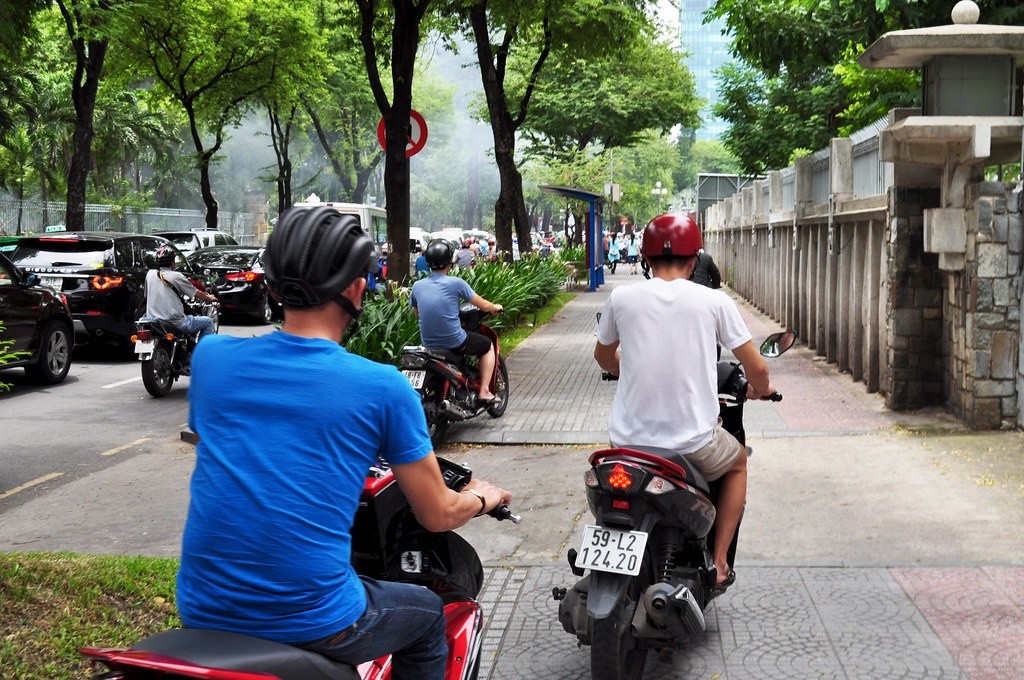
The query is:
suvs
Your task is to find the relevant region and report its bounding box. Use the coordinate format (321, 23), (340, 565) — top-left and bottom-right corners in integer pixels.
(0, 251), (76, 386)
(146, 227), (241, 257)
(9, 230), (220, 362)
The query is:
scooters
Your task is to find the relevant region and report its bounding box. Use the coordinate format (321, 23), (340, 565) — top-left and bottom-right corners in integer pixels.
(76, 426), (523, 680)
(551, 311), (797, 680)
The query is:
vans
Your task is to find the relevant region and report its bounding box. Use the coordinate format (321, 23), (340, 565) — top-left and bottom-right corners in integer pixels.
(292, 200), (421, 258)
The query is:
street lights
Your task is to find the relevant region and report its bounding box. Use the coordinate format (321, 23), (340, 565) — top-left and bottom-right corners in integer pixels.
(650, 181), (668, 215)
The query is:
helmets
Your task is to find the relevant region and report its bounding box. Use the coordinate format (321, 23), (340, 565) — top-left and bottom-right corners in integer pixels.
(423, 238), (455, 269)
(382, 243), (388, 253)
(642, 215), (702, 255)
(263, 206), (379, 307)
(462, 235), (494, 247)
(155, 246), (176, 262)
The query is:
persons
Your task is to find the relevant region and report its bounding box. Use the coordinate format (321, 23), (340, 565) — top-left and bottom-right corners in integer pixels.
(594, 214), (779, 587)
(367, 237), (496, 292)
(176, 203), (512, 680)
(143, 245), (217, 342)
(689, 252), (721, 289)
(410, 238), (503, 404)
(607, 230), (650, 279)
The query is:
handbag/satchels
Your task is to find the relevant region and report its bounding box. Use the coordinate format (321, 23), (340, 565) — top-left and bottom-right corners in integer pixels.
(184, 301), (197, 316)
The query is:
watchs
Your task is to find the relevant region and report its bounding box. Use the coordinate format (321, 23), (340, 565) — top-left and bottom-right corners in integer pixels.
(465, 489), (485, 517)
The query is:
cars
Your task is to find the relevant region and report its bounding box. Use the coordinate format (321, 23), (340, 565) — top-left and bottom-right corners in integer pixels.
(0, 225), (67, 279)
(409, 225), (567, 279)
(186, 244), (282, 324)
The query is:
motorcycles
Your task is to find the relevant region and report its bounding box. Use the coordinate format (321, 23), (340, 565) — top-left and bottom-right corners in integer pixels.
(396, 302), (510, 453)
(130, 268), (222, 397)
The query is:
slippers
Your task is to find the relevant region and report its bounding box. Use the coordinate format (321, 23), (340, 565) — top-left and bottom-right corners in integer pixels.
(477, 393), (502, 404)
(717, 567), (737, 589)
